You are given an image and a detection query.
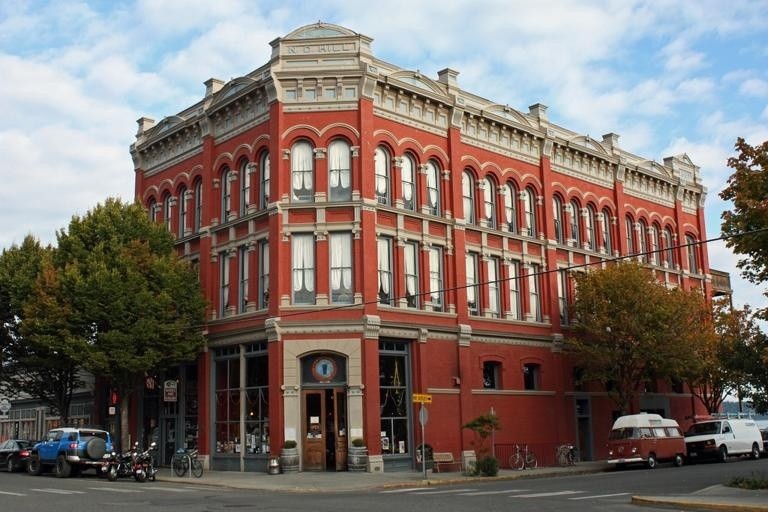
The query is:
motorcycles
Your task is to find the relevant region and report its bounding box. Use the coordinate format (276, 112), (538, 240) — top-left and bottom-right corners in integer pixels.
(101, 441), (158, 481)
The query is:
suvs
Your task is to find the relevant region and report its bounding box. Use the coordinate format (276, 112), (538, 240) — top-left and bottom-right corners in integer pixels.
(27, 427), (115, 478)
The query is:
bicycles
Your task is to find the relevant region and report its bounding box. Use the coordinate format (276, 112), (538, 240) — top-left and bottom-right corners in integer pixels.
(172, 448), (203, 478)
(558, 443), (580, 467)
(509, 441), (538, 471)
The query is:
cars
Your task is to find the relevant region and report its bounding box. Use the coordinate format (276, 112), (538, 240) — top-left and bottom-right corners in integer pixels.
(0, 438), (52, 473)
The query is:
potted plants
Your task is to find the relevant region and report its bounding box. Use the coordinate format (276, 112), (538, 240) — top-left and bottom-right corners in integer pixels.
(348, 439), (367, 470)
(280, 440), (298, 473)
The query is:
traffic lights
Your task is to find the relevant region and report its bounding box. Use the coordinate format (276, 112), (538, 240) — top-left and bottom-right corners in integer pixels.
(111, 390), (117, 406)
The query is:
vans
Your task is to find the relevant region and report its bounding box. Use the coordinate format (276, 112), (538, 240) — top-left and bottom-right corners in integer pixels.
(607, 413), (689, 468)
(685, 418), (765, 462)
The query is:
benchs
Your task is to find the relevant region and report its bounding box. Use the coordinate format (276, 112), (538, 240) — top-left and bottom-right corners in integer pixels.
(418, 453), (462, 473)
